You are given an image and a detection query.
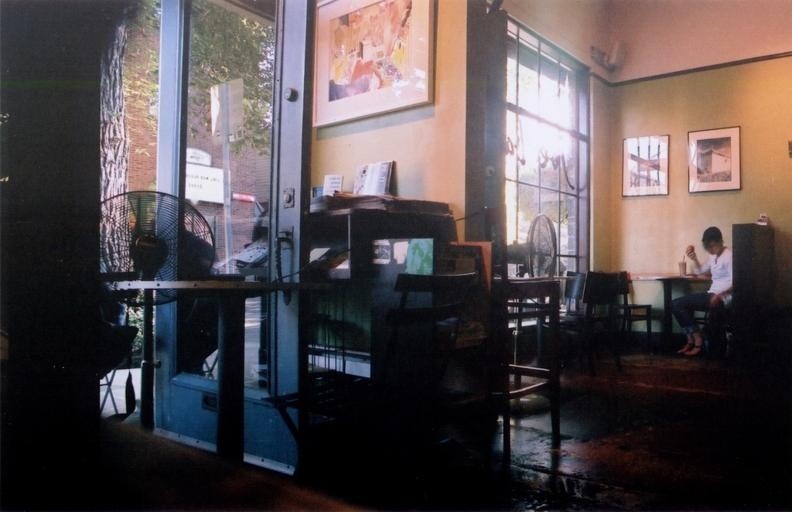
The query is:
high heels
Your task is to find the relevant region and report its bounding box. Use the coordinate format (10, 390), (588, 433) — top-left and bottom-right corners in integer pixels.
(676, 343), (706, 358)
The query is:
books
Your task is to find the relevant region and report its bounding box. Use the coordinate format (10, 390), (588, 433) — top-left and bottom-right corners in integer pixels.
(353, 160), (394, 195)
(313, 174), (343, 198)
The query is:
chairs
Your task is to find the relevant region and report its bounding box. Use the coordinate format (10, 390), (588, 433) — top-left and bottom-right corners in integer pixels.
(508, 269), (652, 372)
(683, 284), (711, 330)
(263, 268), (561, 476)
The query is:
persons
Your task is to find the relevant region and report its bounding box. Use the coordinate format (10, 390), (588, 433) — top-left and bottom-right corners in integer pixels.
(670, 226), (733, 359)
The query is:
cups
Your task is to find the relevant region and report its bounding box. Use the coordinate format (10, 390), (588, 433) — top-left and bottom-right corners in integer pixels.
(680, 261), (686, 278)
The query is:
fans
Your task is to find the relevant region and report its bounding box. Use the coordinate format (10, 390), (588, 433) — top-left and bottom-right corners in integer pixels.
(73, 190), (216, 434)
(506, 212), (558, 365)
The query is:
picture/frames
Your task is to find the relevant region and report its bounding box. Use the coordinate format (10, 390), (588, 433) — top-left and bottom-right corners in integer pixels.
(687, 126), (741, 194)
(621, 135), (670, 197)
(311, 0), (437, 128)
